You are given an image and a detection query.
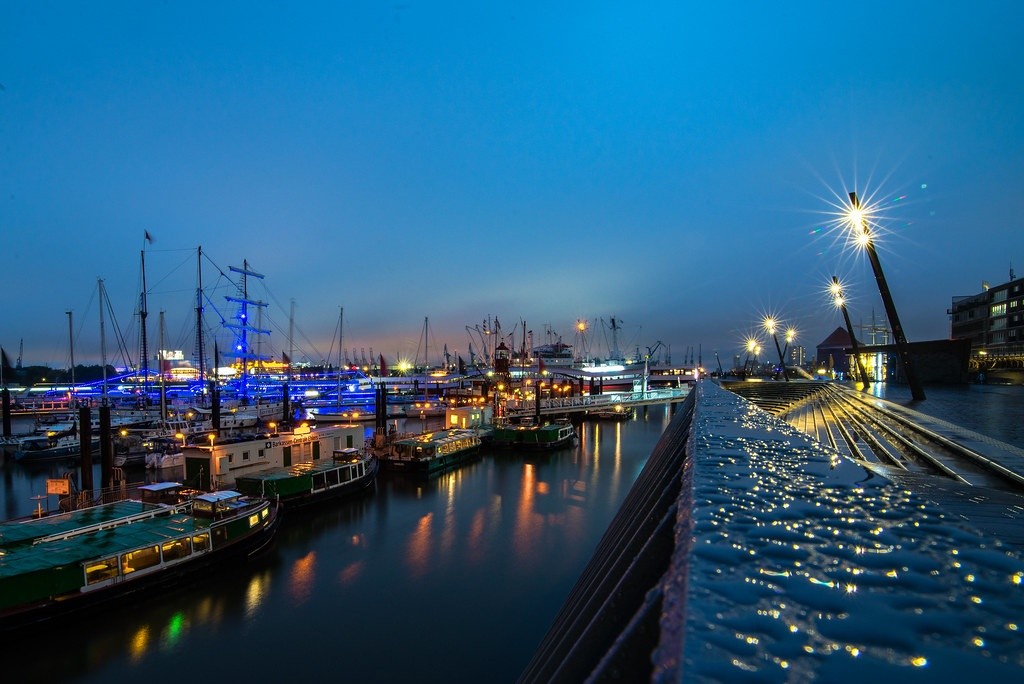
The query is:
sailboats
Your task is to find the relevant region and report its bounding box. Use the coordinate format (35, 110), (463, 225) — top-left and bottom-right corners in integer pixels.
(0, 242), (701, 452)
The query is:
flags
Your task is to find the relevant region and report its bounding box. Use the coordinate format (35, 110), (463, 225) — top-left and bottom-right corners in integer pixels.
(157, 352), (173, 381)
(379, 352), (388, 377)
(282, 350), (291, 371)
(538, 358), (546, 375)
(982, 283), (988, 288)
(458, 353), (469, 377)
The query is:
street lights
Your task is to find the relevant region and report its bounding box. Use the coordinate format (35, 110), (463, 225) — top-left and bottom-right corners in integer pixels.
(831, 277), (872, 388)
(715, 351), (726, 378)
(742, 339), (753, 381)
(767, 315), (790, 382)
(775, 328), (793, 381)
(747, 346), (759, 378)
(848, 190), (928, 402)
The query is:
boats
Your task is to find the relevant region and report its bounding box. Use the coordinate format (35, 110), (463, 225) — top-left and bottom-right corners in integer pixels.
(1, 420), (371, 622)
(362, 385), (575, 472)
